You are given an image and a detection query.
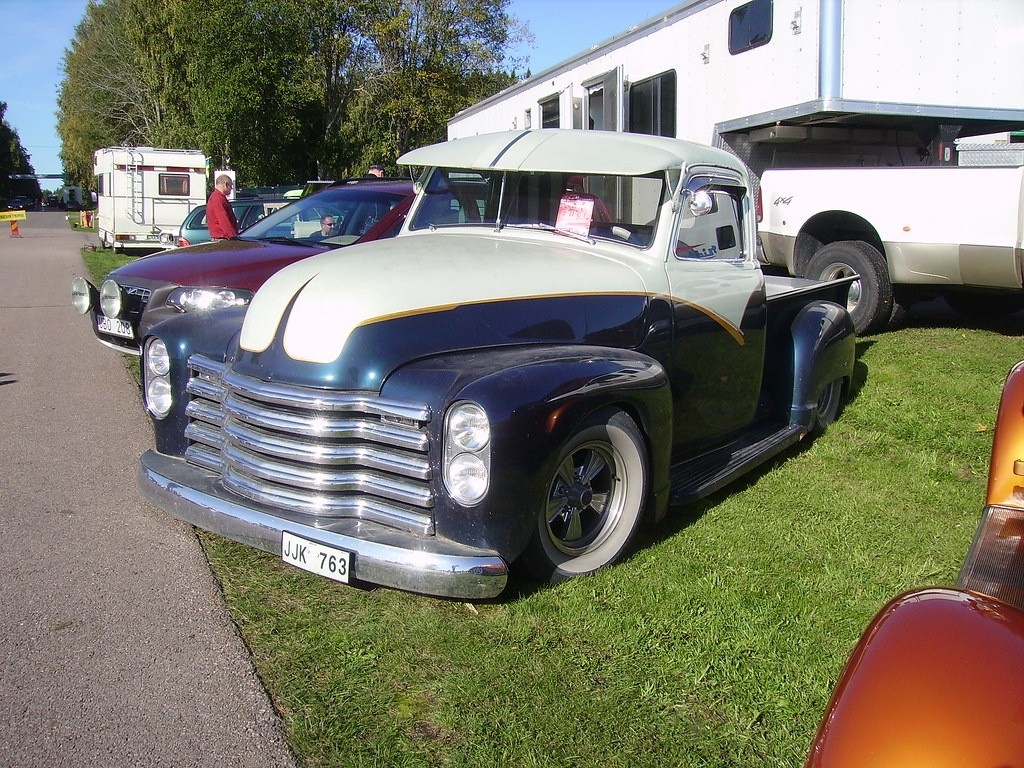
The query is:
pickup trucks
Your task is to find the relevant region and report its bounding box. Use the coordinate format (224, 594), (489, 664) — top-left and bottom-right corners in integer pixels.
(757, 166), (1023, 336)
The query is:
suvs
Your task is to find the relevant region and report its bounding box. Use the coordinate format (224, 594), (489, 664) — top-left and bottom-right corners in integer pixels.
(16, 196), (27, 210)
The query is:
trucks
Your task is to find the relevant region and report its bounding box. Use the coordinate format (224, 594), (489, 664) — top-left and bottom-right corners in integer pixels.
(91, 146), (207, 253)
(63, 185), (82, 211)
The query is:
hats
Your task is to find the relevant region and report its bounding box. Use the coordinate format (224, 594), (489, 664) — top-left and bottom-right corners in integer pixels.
(369, 165), (385, 170)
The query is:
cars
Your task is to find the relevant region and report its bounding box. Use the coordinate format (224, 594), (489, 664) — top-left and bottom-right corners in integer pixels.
(8, 199), (23, 209)
(802, 356), (1024, 768)
(72, 178), (485, 357)
(136, 128), (861, 598)
(176, 187), (305, 247)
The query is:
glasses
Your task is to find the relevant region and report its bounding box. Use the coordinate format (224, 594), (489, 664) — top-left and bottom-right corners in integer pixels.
(324, 223), (335, 226)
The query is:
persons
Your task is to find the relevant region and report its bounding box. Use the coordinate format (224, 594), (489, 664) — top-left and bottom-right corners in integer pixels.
(206, 174), (239, 241)
(35, 198), (45, 211)
(360, 164), (399, 238)
(310, 214), (335, 237)
